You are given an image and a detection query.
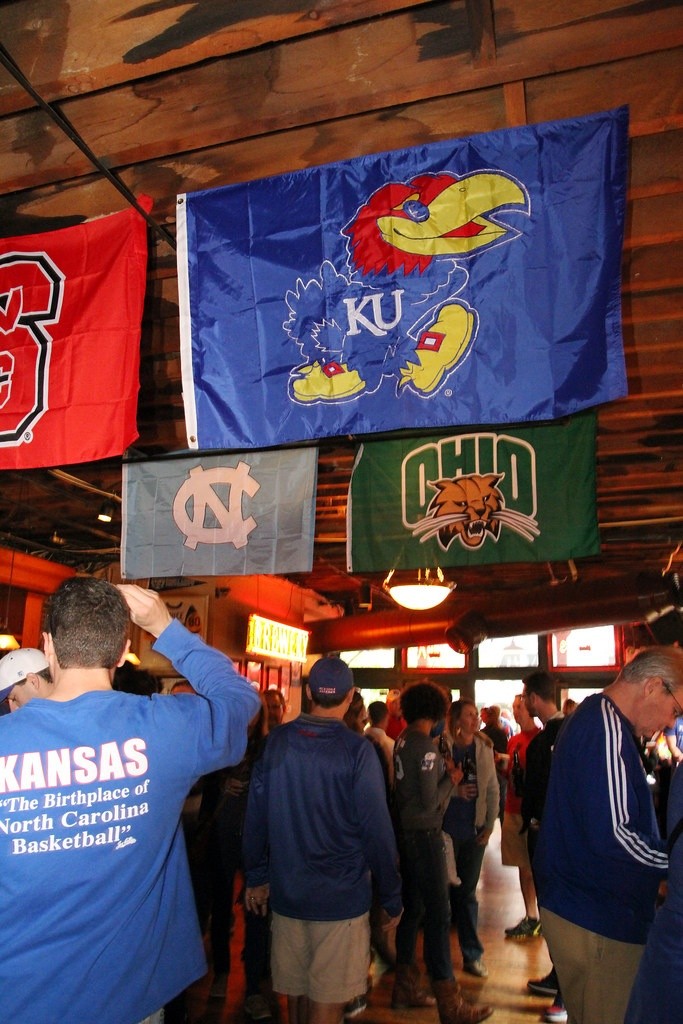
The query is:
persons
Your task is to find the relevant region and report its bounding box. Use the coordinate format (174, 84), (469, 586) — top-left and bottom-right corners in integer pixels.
(0, 575), (683, 1024)
(243, 657), (404, 1024)
(389, 680), (495, 1024)
(538, 649), (683, 1024)
(0, 574), (262, 1024)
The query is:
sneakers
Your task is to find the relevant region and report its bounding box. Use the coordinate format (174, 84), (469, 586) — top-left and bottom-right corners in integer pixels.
(504, 915), (542, 939)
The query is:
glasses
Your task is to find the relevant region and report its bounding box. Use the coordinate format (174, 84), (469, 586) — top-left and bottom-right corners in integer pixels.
(662, 681), (683, 719)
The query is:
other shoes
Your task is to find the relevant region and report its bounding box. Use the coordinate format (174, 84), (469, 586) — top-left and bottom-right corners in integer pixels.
(527, 967), (568, 1022)
(245, 993), (272, 1020)
(344, 998), (366, 1018)
(209, 974), (228, 998)
(462, 957), (487, 977)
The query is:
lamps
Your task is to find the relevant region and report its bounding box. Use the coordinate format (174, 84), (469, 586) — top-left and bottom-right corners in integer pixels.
(98, 490), (119, 522)
(358, 582), (372, 608)
(347, 433), (457, 611)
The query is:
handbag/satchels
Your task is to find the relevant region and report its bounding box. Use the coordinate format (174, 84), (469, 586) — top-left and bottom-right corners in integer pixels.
(439, 828), (462, 887)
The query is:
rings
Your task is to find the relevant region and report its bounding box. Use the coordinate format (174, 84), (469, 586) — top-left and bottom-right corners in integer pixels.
(250, 897), (256, 903)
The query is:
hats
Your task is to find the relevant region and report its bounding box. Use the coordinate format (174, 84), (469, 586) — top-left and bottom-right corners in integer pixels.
(0, 648), (49, 705)
(308, 657), (354, 697)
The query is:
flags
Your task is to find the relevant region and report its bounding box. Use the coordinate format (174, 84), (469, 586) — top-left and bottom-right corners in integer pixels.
(119, 443), (319, 580)
(346, 410), (603, 576)
(1, 193), (156, 468)
(175, 102), (633, 451)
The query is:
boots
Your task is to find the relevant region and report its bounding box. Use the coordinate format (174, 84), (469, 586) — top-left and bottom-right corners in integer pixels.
(391, 967), (436, 1008)
(436, 978), (493, 1024)
